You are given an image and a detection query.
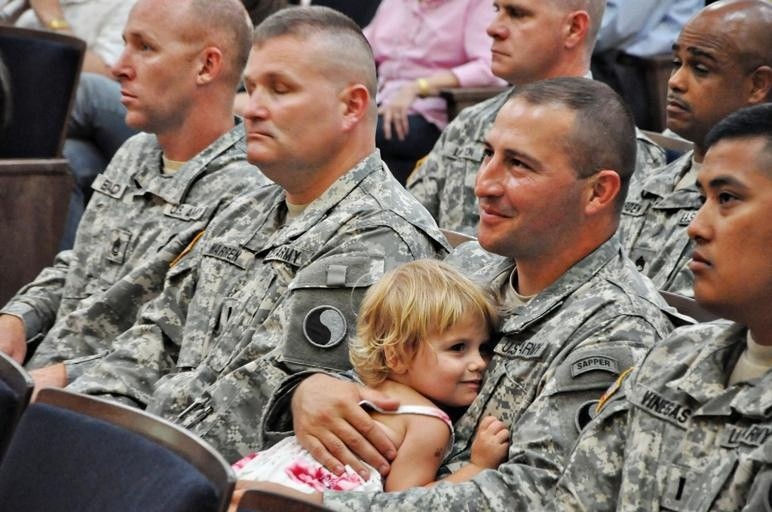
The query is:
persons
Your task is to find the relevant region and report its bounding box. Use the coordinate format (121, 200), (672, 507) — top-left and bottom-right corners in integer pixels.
(220, 259), (509, 511)
(535, 105), (772, 512)
(0, 1), (261, 403)
(356, 1), (509, 172)
(607, 2), (771, 296)
(406, 0), (610, 232)
(0, 0), (180, 257)
(228, 75), (671, 511)
(591, 0), (705, 124)
(71, 5), (452, 470)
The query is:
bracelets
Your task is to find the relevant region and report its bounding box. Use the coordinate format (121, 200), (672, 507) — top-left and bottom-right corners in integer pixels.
(45, 21), (73, 32)
(416, 77), (430, 96)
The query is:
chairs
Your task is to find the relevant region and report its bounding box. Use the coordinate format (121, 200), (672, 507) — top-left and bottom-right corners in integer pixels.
(0, 350), (38, 469)
(440, 79), (512, 131)
(604, 48), (673, 141)
(232, 476), (337, 511)
(1, 20), (89, 322)
(0, 384), (237, 512)
